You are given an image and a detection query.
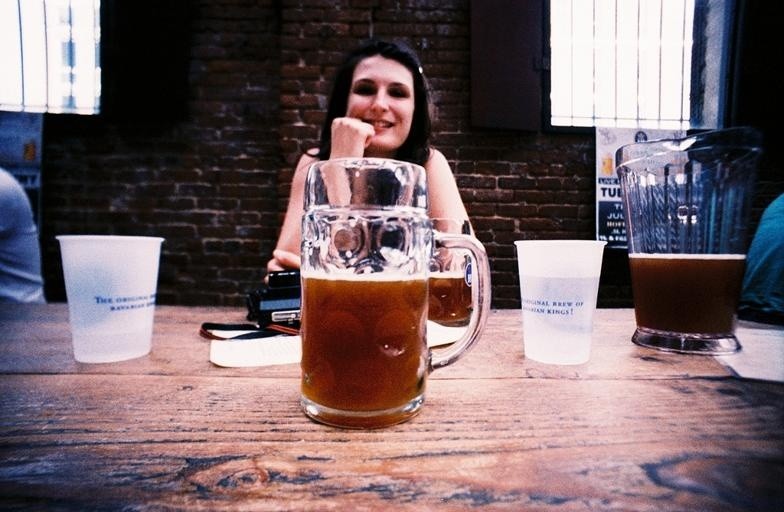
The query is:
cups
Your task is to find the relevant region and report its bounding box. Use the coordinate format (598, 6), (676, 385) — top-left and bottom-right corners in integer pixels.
(513, 239), (610, 367)
(615, 122), (765, 353)
(54, 233), (166, 364)
(297, 158), (493, 430)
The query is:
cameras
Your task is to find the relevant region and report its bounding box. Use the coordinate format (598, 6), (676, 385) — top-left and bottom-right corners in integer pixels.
(247, 270), (303, 324)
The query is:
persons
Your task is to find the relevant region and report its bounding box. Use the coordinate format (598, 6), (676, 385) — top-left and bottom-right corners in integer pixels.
(0, 166), (46, 305)
(736, 190), (783, 328)
(262, 36), (480, 285)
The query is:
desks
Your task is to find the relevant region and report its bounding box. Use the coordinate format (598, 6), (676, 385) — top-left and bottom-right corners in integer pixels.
(0, 301), (783, 512)
